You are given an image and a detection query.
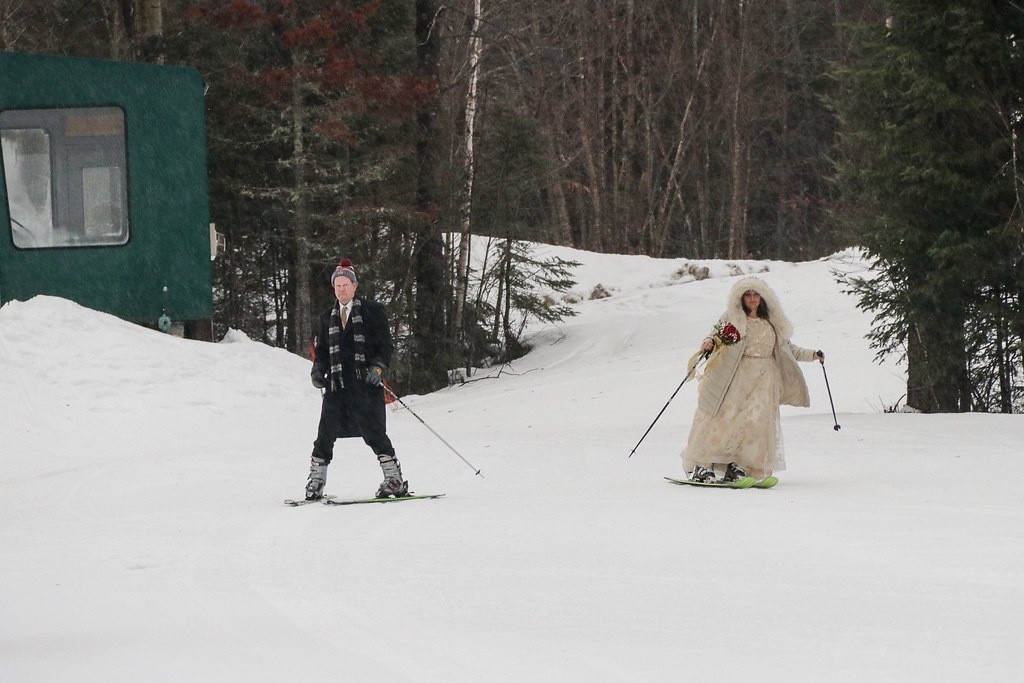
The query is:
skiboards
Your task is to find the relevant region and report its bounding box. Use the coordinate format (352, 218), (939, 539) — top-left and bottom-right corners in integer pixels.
(281, 493), (447, 507)
(664, 475), (780, 489)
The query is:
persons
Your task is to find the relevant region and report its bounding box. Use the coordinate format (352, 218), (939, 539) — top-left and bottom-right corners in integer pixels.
(679, 276), (825, 483)
(305, 258), (411, 500)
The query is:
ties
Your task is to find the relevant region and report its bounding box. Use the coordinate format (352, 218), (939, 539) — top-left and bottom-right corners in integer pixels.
(341, 306), (348, 330)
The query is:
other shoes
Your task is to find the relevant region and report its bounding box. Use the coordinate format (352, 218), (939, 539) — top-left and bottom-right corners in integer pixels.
(691, 465), (715, 482)
(723, 462), (745, 482)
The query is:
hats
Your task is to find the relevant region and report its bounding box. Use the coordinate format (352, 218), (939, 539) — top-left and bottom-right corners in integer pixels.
(331, 259), (357, 287)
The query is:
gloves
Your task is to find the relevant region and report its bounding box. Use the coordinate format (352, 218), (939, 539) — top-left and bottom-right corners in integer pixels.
(366, 367), (383, 387)
(311, 365), (328, 387)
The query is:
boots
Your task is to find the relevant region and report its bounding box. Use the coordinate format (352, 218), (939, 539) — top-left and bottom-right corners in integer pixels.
(375, 453), (404, 499)
(306, 456), (331, 500)
